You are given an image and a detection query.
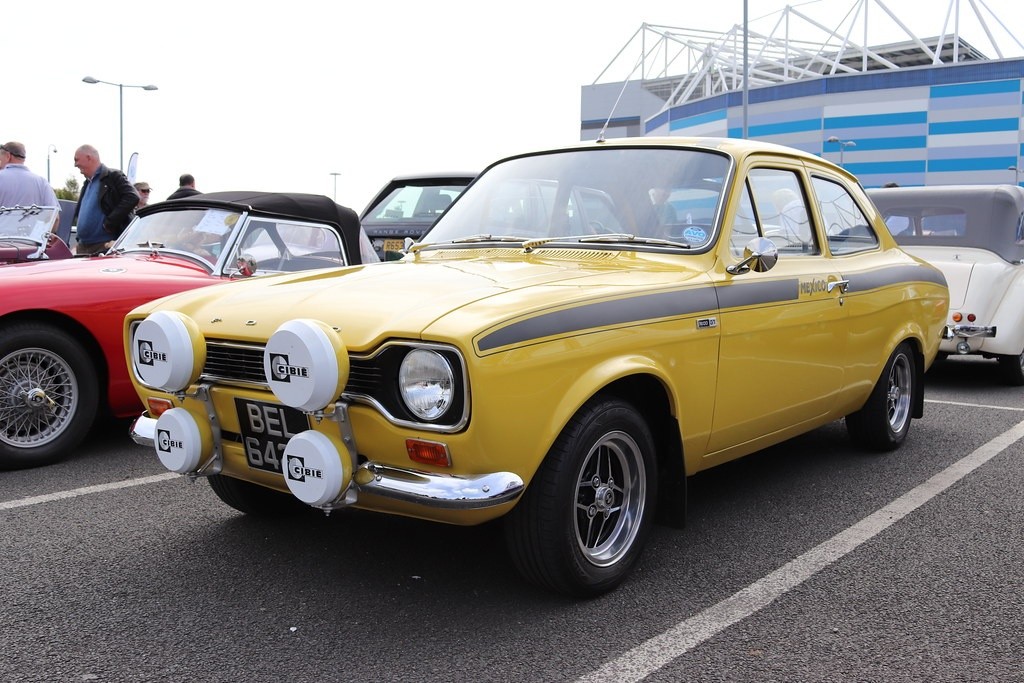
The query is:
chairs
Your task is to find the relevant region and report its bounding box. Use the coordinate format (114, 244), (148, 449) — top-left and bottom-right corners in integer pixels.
(431, 194), (451, 216)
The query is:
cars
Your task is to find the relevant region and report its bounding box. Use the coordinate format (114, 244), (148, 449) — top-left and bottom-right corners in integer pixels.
(0, 172), (786, 472)
(123, 136), (950, 603)
(860, 183), (1024, 385)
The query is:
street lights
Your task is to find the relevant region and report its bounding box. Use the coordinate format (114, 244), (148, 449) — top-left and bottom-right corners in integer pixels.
(47, 144), (57, 185)
(828, 136), (857, 169)
(82, 76), (160, 172)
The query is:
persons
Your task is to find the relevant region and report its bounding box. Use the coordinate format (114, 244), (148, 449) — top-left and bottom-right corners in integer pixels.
(650, 188), (677, 224)
(133, 182), (153, 213)
(167, 174), (203, 202)
(772, 190), (837, 241)
(73, 145), (140, 254)
(0, 141), (63, 234)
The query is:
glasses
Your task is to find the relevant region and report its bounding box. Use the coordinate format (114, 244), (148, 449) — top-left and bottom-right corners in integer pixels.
(1, 142), (26, 159)
(138, 189), (149, 194)
